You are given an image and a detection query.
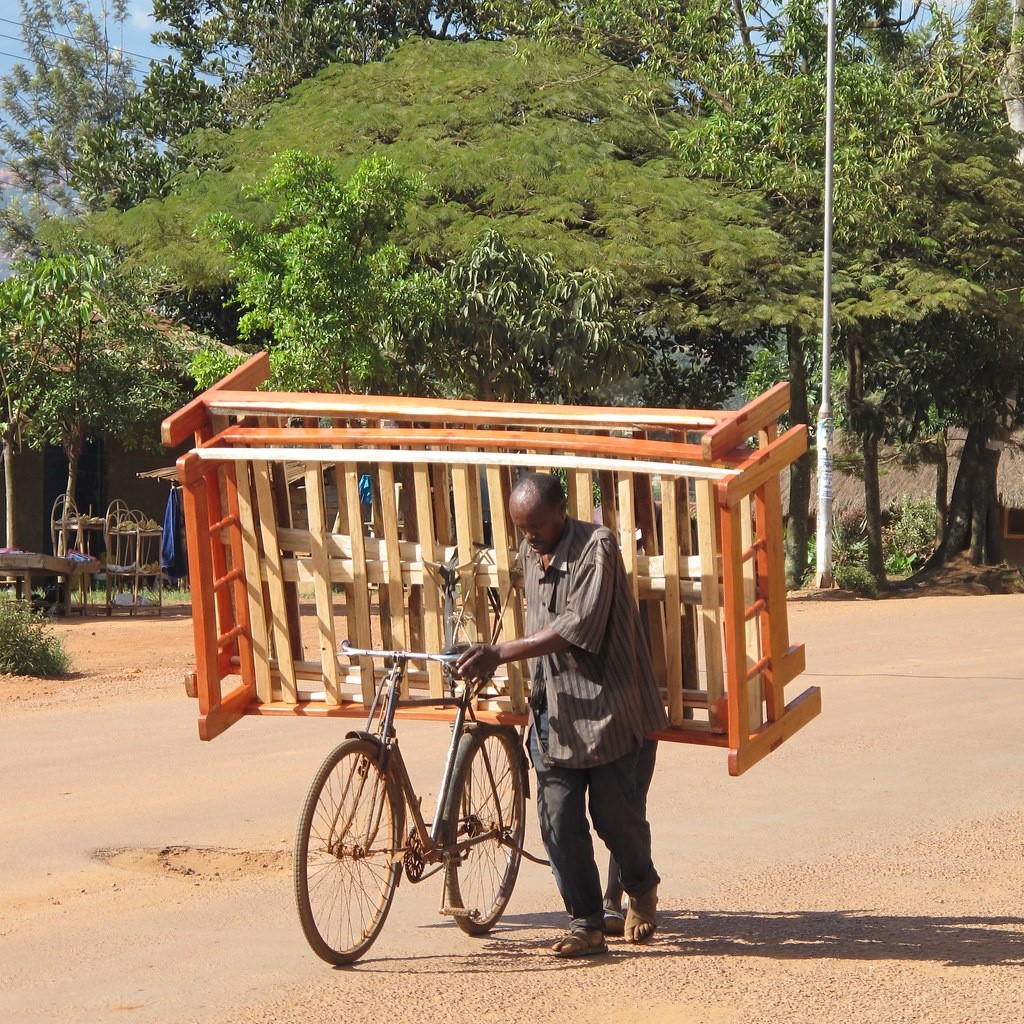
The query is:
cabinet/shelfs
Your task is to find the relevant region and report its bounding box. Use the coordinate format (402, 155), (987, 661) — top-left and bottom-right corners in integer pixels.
(50, 493), (164, 618)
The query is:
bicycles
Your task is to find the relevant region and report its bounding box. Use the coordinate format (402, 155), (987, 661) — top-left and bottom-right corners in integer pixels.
(296, 640), (554, 965)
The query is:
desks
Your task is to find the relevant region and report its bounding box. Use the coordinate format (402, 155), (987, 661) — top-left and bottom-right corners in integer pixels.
(0, 568), (70, 619)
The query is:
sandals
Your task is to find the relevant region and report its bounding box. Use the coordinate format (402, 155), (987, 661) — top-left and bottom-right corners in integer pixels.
(623, 895), (657, 942)
(553, 927), (608, 957)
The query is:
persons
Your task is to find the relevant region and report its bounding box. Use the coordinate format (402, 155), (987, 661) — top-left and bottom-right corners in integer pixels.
(590, 452), (663, 663)
(456, 473), (670, 958)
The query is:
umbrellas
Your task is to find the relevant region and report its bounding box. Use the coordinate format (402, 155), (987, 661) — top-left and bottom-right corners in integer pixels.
(158, 482), (189, 593)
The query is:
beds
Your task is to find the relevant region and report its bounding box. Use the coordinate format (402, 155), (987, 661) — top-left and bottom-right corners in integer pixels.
(163, 350), (821, 779)
